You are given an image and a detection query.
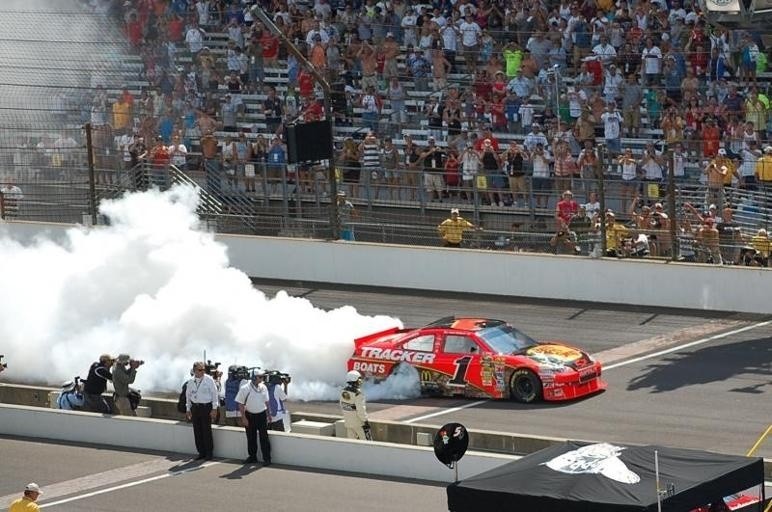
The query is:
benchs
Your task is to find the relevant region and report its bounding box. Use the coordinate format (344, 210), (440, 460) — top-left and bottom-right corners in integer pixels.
(69, 25), (772, 216)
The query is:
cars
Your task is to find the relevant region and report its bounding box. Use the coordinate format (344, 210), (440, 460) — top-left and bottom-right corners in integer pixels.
(347, 314), (608, 403)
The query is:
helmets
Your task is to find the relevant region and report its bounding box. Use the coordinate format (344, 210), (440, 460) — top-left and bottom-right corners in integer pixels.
(345, 370), (362, 382)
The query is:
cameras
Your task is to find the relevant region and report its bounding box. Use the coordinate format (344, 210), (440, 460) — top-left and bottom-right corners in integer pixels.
(130, 360), (144, 369)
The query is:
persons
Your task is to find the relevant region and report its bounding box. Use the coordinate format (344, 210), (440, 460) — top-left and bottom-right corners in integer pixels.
(233, 368), (273, 468)
(2, 85), (111, 223)
(83, 353), (117, 413)
(224, 365), (244, 427)
(238, 374), (268, 391)
(111, 353), (140, 416)
(106, 1), (772, 266)
(7, 482), (44, 512)
(177, 370), (224, 424)
(339, 370), (374, 440)
(56, 380), (84, 410)
(267, 371), (292, 433)
(0, 363), (8, 374)
(186, 360), (220, 462)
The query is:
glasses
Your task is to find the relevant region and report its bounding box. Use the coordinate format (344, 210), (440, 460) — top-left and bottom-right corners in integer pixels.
(196, 368), (206, 372)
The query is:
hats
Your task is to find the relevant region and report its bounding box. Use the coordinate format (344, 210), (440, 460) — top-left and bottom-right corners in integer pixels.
(335, 32), (772, 216)
(63, 381), (76, 392)
(24, 483), (43, 495)
(250, 369), (266, 378)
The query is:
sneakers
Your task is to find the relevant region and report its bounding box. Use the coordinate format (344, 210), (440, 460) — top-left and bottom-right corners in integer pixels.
(242, 455), (271, 467)
(195, 454), (213, 461)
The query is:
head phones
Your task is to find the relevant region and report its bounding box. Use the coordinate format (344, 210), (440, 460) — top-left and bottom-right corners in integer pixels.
(251, 368), (257, 383)
